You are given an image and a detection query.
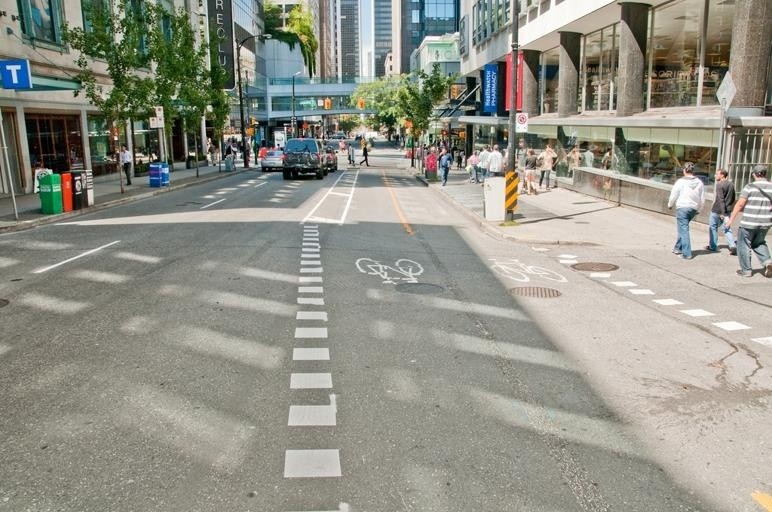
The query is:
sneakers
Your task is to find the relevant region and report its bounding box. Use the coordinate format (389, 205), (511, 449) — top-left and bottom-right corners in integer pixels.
(729, 246), (737, 256)
(671, 246), (694, 260)
(705, 245), (720, 252)
(735, 267), (754, 277)
(764, 262), (772, 278)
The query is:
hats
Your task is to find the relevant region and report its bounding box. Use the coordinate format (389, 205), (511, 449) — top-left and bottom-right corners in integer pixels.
(679, 161), (696, 170)
(749, 165), (768, 173)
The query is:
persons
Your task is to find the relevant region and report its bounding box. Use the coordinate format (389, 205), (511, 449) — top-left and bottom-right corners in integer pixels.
(347, 143), (355, 166)
(360, 137), (366, 149)
(338, 137), (345, 155)
(666, 161), (705, 260)
(723, 165), (772, 278)
(360, 145), (369, 167)
(406, 134), (561, 197)
(564, 141), (621, 183)
(205, 134), (283, 167)
(118, 142), (133, 185)
(705, 168), (738, 255)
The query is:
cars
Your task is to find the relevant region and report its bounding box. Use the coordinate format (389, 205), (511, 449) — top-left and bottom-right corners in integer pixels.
(261, 131), (375, 180)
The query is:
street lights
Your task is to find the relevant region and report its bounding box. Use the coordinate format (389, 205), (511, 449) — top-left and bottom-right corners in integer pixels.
(292, 71), (302, 137)
(235, 32), (272, 169)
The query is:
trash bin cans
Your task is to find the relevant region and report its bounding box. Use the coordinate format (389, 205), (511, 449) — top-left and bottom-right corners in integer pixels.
(187, 156), (195, 169)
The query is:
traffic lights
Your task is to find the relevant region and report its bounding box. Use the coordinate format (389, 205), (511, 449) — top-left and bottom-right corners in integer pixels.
(249, 128), (254, 135)
(442, 131), (447, 135)
(250, 117), (255, 125)
